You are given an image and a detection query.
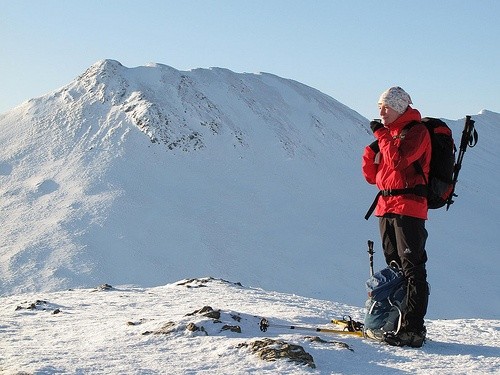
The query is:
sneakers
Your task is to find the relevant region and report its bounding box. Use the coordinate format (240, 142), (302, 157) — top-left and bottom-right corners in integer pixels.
(381, 323), (427, 348)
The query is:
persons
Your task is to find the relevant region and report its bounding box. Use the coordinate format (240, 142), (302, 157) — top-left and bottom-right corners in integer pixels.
(362, 83), (432, 347)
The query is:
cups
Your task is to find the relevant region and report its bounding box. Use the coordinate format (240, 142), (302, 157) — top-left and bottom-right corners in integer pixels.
(373, 119), (381, 124)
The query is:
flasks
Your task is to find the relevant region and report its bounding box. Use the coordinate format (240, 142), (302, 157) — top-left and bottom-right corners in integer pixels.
(373, 151), (380, 164)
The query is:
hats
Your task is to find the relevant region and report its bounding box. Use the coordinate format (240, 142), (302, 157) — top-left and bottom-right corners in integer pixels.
(378, 86), (413, 115)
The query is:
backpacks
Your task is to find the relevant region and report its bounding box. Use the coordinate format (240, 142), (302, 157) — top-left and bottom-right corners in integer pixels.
(364, 117), (457, 221)
(363, 260), (405, 340)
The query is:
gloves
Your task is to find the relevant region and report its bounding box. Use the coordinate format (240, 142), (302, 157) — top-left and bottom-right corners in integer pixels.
(369, 140), (380, 154)
(369, 121), (384, 133)
